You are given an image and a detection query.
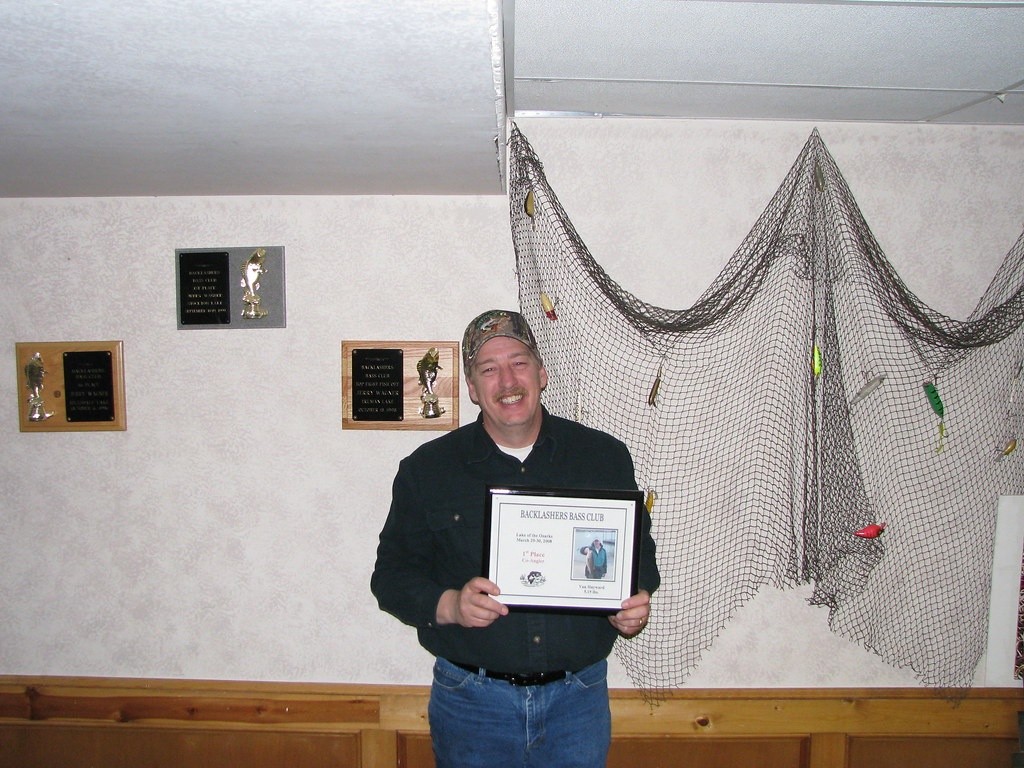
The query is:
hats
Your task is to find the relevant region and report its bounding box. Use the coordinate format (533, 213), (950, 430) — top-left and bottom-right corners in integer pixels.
(462, 310), (537, 366)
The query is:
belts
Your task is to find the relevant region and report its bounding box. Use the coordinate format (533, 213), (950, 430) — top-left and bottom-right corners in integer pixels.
(446, 658), (566, 688)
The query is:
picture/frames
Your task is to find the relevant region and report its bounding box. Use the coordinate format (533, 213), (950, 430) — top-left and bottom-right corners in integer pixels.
(341, 339), (460, 430)
(481, 485), (644, 618)
(16, 341), (128, 433)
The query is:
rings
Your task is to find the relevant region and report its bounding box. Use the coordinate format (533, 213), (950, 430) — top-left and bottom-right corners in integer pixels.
(640, 619), (643, 625)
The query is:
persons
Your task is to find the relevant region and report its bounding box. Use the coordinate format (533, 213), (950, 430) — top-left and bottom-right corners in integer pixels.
(370, 310), (661, 768)
(580, 537), (608, 578)
